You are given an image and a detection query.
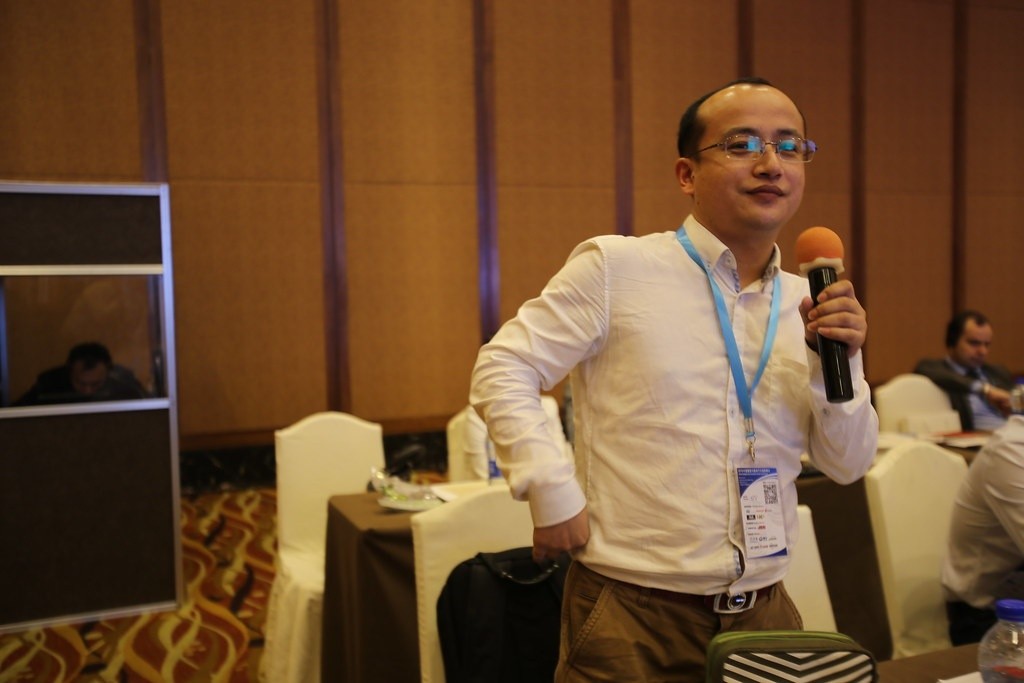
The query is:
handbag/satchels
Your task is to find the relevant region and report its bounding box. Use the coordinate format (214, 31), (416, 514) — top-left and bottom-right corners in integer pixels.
(436, 546), (572, 683)
(705, 630), (879, 683)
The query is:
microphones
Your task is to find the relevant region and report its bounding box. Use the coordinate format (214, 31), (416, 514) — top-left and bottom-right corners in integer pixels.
(797, 226), (853, 402)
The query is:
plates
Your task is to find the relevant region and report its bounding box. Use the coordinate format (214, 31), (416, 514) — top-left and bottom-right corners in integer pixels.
(379, 491), (444, 511)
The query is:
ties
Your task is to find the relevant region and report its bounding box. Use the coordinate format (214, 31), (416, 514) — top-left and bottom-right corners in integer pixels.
(967, 370), (1007, 418)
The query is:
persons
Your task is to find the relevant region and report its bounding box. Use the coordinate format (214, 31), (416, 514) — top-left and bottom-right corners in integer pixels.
(913, 310), (1024, 433)
(9, 343), (149, 408)
(469, 78), (880, 683)
(942, 414), (1024, 646)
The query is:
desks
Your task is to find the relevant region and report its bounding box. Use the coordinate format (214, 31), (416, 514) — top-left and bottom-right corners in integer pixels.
(319, 432), (995, 683)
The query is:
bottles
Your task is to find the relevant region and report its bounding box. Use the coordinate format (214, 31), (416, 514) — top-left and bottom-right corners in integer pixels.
(486, 434), (505, 485)
(976, 601), (1024, 683)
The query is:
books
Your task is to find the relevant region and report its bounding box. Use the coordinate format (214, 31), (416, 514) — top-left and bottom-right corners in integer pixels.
(923, 431), (993, 448)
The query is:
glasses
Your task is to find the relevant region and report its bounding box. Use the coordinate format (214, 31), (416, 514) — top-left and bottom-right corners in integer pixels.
(688, 135), (818, 163)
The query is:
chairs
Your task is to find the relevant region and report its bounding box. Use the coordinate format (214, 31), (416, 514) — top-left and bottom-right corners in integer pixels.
(259, 411), (386, 683)
(864, 440), (968, 661)
(447, 395), (558, 483)
(782, 503), (840, 632)
(873, 372), (952, 441)
(409, 483), (533, 683)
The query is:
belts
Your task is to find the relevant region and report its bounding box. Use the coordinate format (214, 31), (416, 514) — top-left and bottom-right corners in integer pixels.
(650, 584), (775, 615)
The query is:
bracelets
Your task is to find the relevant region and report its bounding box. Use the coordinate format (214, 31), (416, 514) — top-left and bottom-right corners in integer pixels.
(981, 381), (990, 398)
